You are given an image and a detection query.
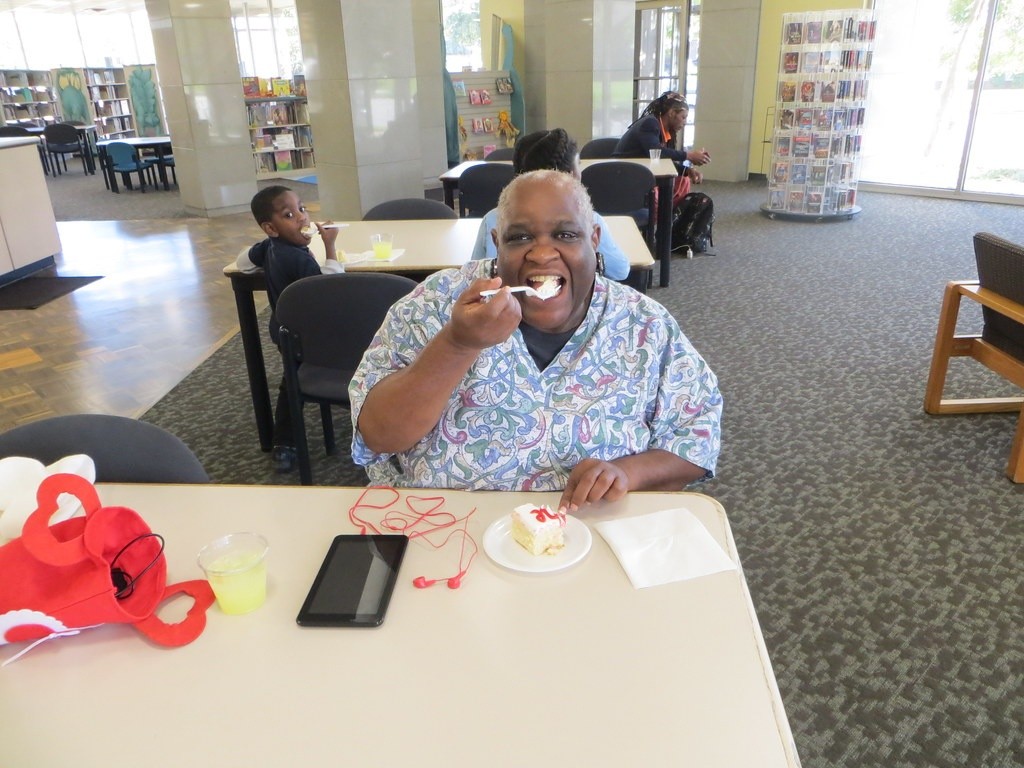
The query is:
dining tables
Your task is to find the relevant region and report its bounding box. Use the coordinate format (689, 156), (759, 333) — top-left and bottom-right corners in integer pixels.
(224, 216), (655, 451)
(0, 484), (802, 768)
(439, 158), (678, 288)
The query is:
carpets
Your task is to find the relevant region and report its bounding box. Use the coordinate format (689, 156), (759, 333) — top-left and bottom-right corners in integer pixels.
(0, 276), (104, 310)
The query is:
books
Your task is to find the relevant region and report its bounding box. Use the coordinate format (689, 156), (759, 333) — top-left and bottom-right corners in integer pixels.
(242, 75), (316, 175)
(84, 69), (131, 141)
(767, 19), (877, 215)
(0, 72), (63, 127)
(452, 77), (514, 159)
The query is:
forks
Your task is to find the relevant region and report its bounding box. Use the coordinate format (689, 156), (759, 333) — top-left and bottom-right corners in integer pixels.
(478, 278), (562, 299)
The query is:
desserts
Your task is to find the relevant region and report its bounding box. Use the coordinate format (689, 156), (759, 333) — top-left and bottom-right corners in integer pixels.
(512, 503), (566, 556)
(301, 226), (311, 233)
(336, 250), (345, 264)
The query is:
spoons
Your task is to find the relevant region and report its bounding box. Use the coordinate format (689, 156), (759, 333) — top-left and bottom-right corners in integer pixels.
(301, 224), (350, 235)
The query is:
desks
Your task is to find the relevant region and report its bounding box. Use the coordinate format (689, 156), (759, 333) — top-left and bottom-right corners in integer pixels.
(0, 136), (62, 287)
(96, 137), (171, 190)
(25, 125), (103, 175)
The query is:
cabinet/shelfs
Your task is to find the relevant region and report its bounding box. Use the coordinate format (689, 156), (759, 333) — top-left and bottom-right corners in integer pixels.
(244, 96), (317, 181)
(0, 69), (54, 127)
(50, 68), (136, 158)
(123, 63), (170, 157)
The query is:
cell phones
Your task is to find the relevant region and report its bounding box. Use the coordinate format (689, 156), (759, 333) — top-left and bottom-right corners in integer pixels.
(296, 535), (409, 627)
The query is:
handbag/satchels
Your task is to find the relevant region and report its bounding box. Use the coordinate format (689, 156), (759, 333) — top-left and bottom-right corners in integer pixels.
(0, 473), (216, 646)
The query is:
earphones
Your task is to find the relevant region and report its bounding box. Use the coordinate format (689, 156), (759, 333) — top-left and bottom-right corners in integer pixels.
(447, 571), (466, 589)
(413, 577), (437, 588)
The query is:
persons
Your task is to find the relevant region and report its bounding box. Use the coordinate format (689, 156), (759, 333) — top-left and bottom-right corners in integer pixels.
(610, 91), (712, 239)
(348, 171), (723, 515)
(469, 128), (631, 281)
(495, 110), (520, 148)
(248, 186), (345, 473)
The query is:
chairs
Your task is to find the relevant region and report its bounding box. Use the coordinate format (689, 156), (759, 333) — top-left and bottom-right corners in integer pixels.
(579, 138), (621, 159)
(0, 414), (210, 485)
(362, 198), (460, 284)
(923, 231), (1024, 484)
(274, 273), (420, 486)
(0, 120), (175, 194)
(458, 164), (514, 218)
(581, 162), (655, 295)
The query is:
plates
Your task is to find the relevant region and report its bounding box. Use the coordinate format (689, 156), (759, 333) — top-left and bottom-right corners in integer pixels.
(342, 253), (368, 264)
(482, 512), (592, 572)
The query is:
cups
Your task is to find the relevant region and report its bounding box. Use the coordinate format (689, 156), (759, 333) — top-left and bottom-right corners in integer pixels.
(196, 531), (270, 615)
(371, 233), (394, 258)
(649, 149), (661, 164)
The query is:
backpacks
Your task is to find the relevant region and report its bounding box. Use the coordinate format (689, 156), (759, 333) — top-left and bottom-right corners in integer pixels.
(673, 192), (714, 254)
